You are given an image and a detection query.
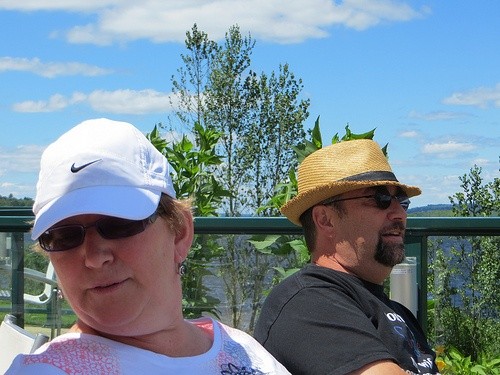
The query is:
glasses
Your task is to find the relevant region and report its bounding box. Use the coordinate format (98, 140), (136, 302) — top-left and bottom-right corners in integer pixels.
(38, 218), (150, 251)
(323, 188), (411, 211)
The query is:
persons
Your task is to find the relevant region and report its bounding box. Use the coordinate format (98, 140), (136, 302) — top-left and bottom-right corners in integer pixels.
(257, 139), (444, 375)
(4, 116), (300, 375)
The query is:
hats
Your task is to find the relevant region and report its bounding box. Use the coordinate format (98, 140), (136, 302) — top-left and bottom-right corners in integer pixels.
(32, 117), (176, 240)
(279, 139), (421, 226)
